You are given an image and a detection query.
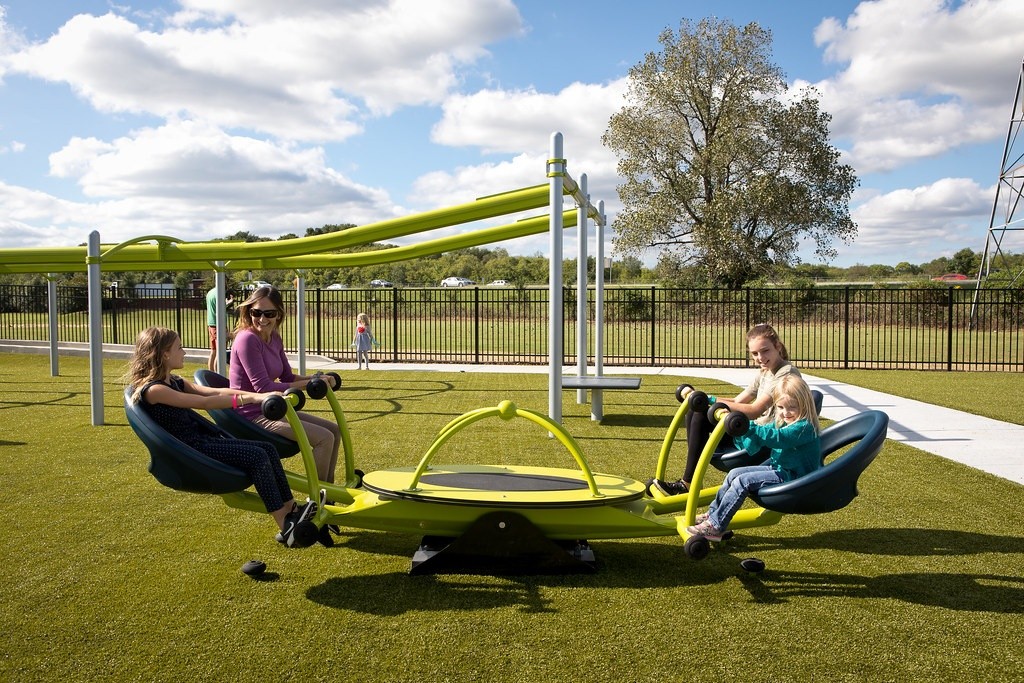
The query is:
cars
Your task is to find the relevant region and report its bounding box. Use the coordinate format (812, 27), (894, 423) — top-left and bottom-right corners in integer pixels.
(367, 279), (394, 288)
(326, 283), (347, 289)
(440, 277), (476, 287)
(249, 281), (271, 289)
(929, 273), (968, 280)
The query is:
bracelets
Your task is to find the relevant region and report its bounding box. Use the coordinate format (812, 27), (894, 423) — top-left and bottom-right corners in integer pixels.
(707, 394), (716, 406)
(232, 394), (237, 410)
(239, 394), (244, 407)
(314, 370), (323, 378)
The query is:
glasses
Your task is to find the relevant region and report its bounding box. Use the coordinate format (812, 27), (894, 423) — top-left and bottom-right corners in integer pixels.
(250, 308), (278, 318)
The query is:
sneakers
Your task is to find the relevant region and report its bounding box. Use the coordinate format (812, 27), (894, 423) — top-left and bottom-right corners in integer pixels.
(280, 500), (317, 548)
(686, 521), (723, 542)
(328, 522), (341, 535)
(684, 512), (710, 525)
(318, 523), (333, 547)
(306, 488), (327, 507)
(653, 478), (688, 496)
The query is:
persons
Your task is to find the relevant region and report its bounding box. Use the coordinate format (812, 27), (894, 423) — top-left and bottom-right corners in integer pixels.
(686, 373), (825, 542)
(652, 323), (804, 498)
(228, 283), (342, 547)
(119, 325), (329, 543)
(350, 314), (382, 371)
(205, 273), (235, 373)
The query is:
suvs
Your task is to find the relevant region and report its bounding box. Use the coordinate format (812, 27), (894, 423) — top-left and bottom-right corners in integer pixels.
(487, 280), (510, 286)
